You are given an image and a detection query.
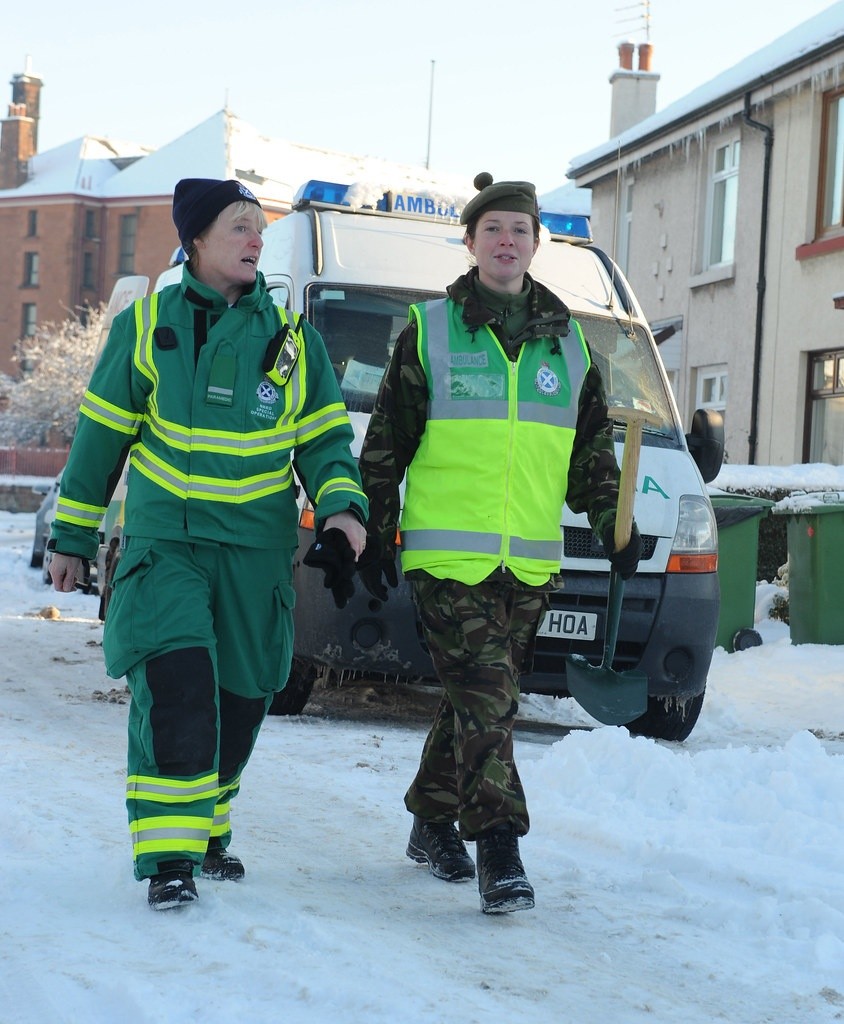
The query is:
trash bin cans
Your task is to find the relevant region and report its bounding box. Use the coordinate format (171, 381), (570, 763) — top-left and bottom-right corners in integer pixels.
(708, 494), (776, 655)
(771, 490), (844, 648)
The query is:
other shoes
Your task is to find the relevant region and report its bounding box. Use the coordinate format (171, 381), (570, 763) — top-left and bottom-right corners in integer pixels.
(148, 872), (198, 910)
(200, 847), (244, 879)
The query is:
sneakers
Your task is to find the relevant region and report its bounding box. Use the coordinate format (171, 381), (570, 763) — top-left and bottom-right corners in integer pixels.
(476, 830), (534, 913)
(406, 814), (476, 881)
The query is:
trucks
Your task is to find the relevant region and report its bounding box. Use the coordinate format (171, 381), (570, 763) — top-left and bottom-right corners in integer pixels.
(91, 180), (724, 742)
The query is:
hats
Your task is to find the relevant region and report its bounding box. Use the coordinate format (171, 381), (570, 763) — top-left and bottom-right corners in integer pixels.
(172, 178), (262, 243)
(459, 172), (540, 227)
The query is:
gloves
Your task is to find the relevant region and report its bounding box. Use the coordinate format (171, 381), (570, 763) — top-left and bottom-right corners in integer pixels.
(356, 539), (398, 602)
(303, 528), (358, 609)
(602, 526), (642, 581)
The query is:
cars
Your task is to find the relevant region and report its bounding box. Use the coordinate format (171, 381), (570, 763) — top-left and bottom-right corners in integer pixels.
(29, 466), (65, 568)
(43, 481), (105, 594)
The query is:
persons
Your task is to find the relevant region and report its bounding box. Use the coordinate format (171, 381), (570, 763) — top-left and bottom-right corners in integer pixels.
(47, 178), (371, 910)
(357, 171), (647, 916)
